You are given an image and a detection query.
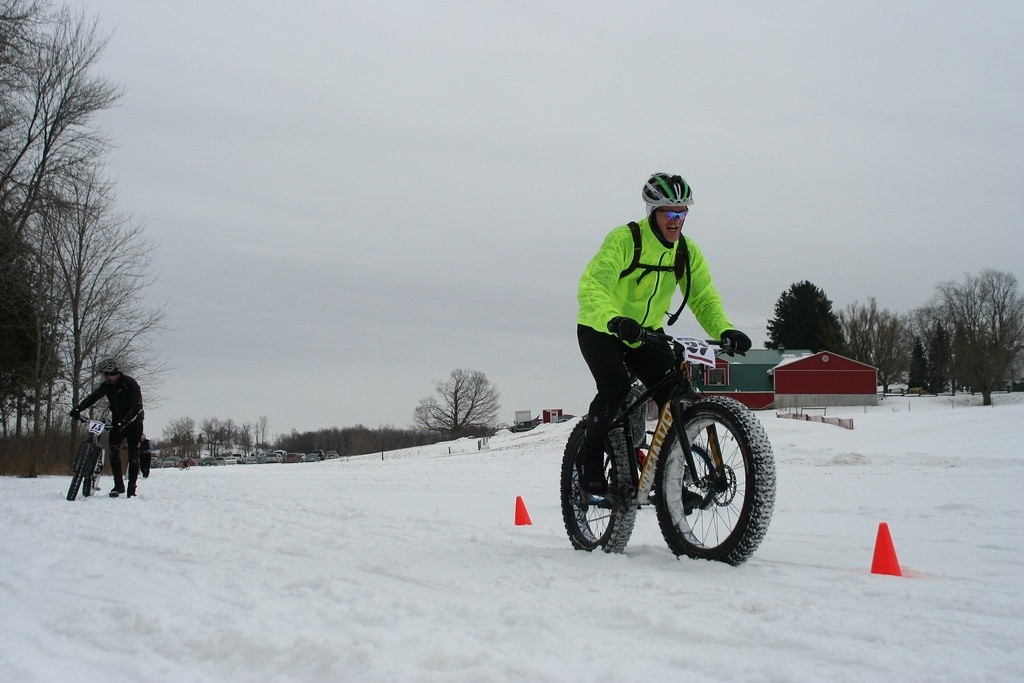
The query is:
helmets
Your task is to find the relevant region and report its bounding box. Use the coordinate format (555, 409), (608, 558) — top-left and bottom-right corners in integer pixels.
(96, 360), (118, 375)
(641, 172), (694, 206)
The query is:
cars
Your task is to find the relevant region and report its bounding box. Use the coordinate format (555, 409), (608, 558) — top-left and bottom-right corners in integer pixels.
(509, 421), (535, 432)
(149, 450), (339, 468)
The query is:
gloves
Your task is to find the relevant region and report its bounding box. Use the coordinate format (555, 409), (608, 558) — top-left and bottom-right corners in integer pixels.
(111, 427), (122, 436)
(607, 316), (646, 345)
(720, 329), (751, 357)
(70, 409), (80, 418)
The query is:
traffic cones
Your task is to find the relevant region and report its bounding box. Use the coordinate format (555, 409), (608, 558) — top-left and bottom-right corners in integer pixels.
(872, 522), (904, 577)
(514, 496), (533, 525)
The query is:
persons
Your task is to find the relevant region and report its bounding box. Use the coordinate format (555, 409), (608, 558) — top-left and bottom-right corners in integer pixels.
(70, 359), (145, 498)
(138, 433), (153, 478)
(574, 173), (753, 508)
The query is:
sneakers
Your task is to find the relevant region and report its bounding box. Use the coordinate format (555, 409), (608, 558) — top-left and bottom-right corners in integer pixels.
(576, 452), (608, 495)
(109, 485), (125, 496)
(681, 485), (702, 507)
(127, 490), (135, 498)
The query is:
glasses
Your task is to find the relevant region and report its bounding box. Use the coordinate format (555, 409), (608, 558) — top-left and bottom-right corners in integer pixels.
(655, 208), (688, 222)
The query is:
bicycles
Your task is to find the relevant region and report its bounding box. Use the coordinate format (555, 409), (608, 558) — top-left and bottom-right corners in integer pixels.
(66, 415), (113, 500)
(560, 336), (778, 568)
(179, 461), (190, 471)
